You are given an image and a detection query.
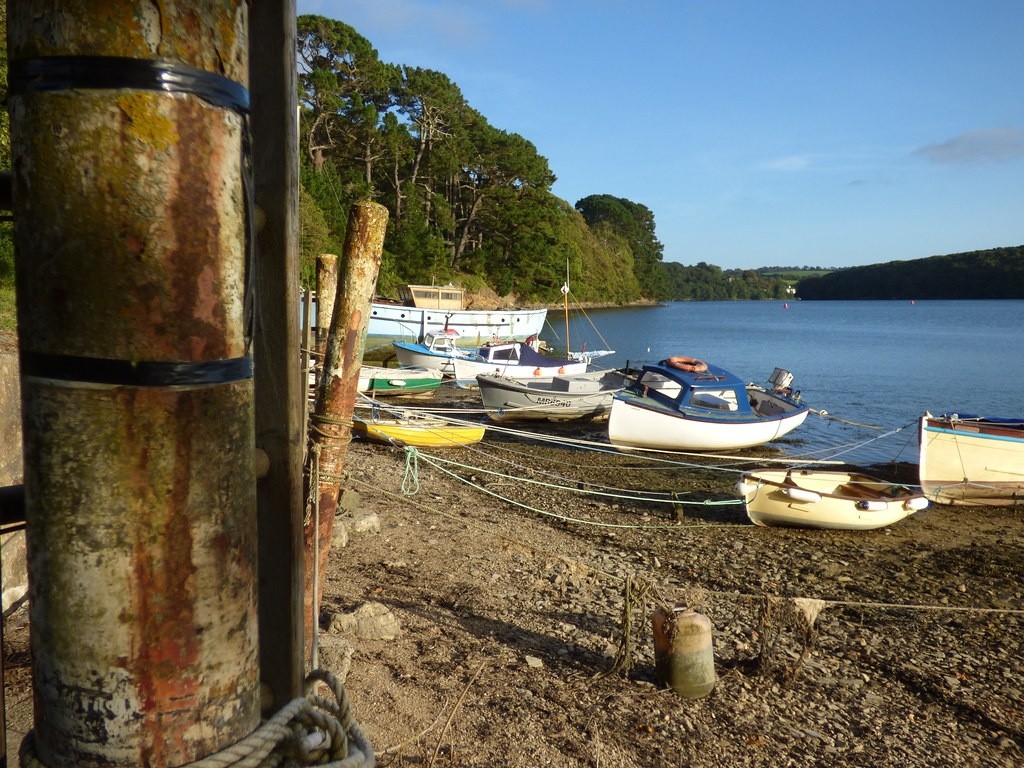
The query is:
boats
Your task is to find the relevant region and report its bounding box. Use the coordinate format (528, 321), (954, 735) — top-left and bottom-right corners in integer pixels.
(607, 357), (812, 453)
(918, 412), (1024, 508)
(742, 465), (931, 531)
(300, 281), (549, 338)
(353, 417), (486, 447)
(302, 358), (443, 396)
(392, 257), (615, 388)
(475, 367), (662, 423)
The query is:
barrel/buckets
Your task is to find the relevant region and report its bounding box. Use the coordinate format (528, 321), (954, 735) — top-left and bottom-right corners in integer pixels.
(655, 612), (715, 699)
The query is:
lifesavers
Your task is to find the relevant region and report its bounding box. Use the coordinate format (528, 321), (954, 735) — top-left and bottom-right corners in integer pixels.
(666, 355), (709, 374)
